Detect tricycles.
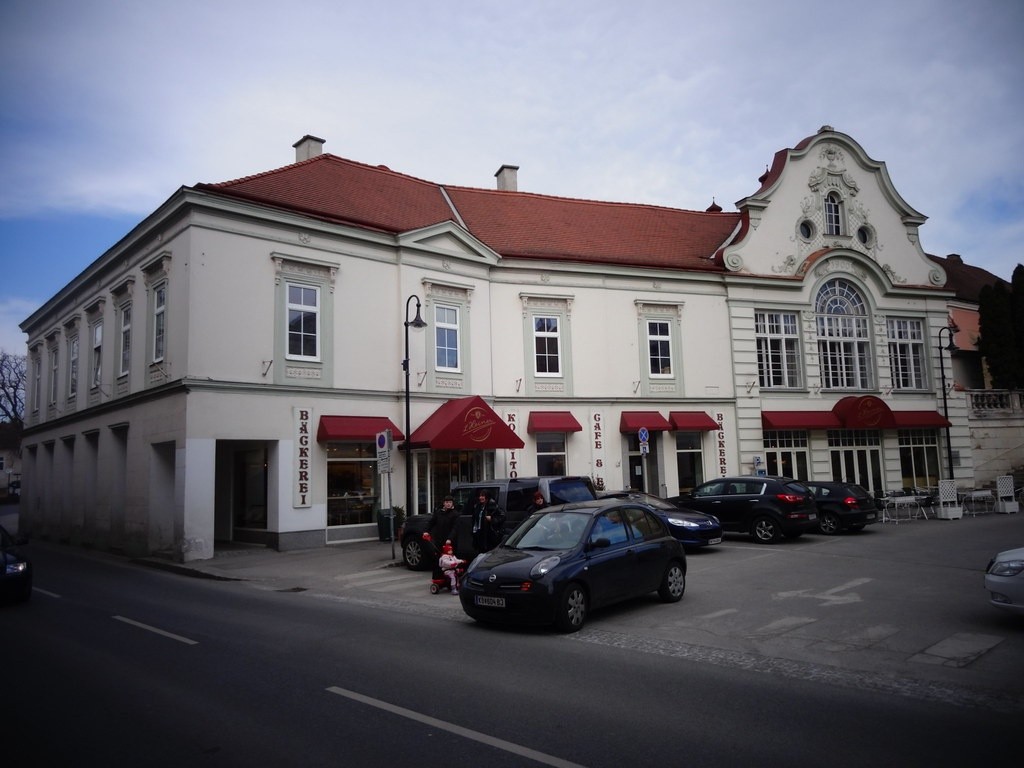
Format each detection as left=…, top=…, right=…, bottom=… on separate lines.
left=420, top=532, right=468, bottom=595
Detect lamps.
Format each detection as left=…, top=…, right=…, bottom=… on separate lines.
left=879, top=387, right=894, bottom=400
left=806, top=387, right=823, bottom=398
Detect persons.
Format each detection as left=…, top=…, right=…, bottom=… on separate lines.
left=528, top=492, right=552, bottom=520
left=429, top=488, right=505, bottom=595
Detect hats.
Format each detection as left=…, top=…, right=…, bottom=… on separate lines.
left=534, top=492, right=542, bottom=501
left=443, top=545, right=452, bottom=554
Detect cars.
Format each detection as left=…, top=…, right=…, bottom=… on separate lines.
left=803, top=480, right=879, bottom=537
left=456, top=497, right=687, bottom=635
left=982, top=547, right=1024, bottom=615
left=596, top=490, right=724, bottom=547
left=0, top=522, right=35, bottom=606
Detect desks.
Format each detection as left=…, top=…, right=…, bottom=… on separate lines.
left=869, top=486, right=997, bottom=525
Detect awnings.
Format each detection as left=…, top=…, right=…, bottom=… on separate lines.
left=761, top=395, right=952, bottom=430
left=317, top=415, right=405, bottom=442
left=526, top=411, right=583, bottom=433
left=398, top=395, right=525, bottom=451
left=619, top=411, right=720, bottom=433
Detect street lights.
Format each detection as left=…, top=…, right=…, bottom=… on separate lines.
left=938, top=325, right=960, bottom=479
left=400, top=292, right=430, bottom=518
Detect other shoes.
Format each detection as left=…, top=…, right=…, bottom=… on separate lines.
left=452, top=590, right=459, bottom=595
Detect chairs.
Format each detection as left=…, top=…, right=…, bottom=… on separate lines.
left=873, top=491, right=895, bottom=521
left=544, top=519, right=591, bottom=545
left=916, top=496, right=935, bottom=521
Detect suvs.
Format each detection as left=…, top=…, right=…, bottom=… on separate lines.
left=397, top=474, right=600, bottom=572
left=664, top=475, right=823, bottom=544
